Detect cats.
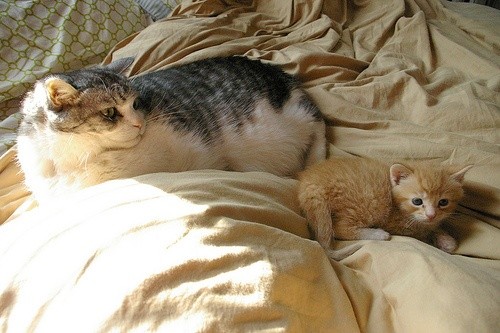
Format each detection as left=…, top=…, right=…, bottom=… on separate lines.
left=297, top=156, right=473, bottom=257
left=15, top=55, right=328, bottom=192
